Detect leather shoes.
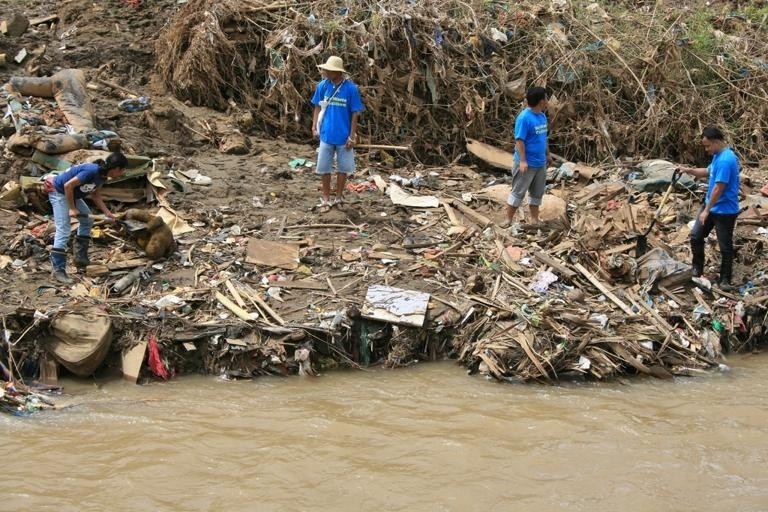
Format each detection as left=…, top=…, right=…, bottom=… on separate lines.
left=334, top=197, right=349, bottom=211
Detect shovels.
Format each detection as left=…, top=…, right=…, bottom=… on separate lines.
left=76, top=213, right=149, bottom=232
left=636, top=169, right=682, bottom=258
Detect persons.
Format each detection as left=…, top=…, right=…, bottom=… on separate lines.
left=47, top=153, right=129, bottom=285
left=677, top=125, right=741, bottom=285
left=310, top=54, right=365, bottom=208
left=501, top=87, right=550, bottom=229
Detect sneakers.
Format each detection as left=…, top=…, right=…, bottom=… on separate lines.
left=687, top=268, right=702, bottom=277
left=718, top=277, right=731, bottom=292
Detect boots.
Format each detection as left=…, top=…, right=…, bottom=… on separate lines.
left=49, top=249, right=75, bottom=284
left=72, top=235, right=103, bottom=266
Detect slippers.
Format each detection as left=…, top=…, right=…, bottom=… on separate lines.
left=320, top=200, right=332, bottom=213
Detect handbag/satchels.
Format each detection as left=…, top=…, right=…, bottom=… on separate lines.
left=315, top=100, right=328, bottom=136
left=42, top=177, right=55, bottom=192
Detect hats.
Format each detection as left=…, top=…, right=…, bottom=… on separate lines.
left=316, top=56, right=346, bottom=75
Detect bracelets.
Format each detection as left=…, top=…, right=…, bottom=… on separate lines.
left=348, top=135, right=355, bottom=143
